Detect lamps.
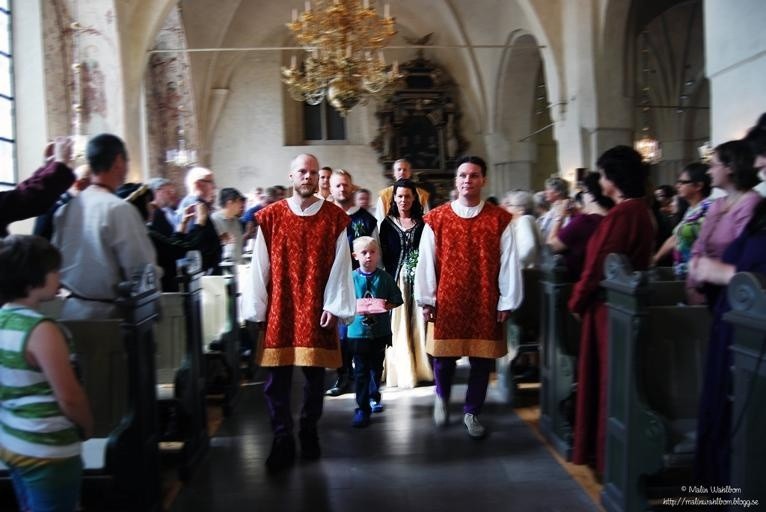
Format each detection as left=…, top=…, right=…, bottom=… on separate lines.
left=281, top=0, right=403, bottom=116
left=165, top=33, right=198, bottom=167
left=633, top=27, right=663, bottom=165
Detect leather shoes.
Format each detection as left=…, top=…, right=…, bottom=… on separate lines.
left=323, top=367, right=353, bottom=397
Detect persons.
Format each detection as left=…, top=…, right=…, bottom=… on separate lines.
left=0, top=109, right=765, bottom=512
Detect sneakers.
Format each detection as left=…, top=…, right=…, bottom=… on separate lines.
left=298, top=425, right=323, bottom=462
left=432, top=391, right=449, bottom=428
left=352, top=407, right=371, bottom=427
left=369, top=398, right=383, bottom=413
left=264, top=431, right=297, bottom=474
left=463, top=413, right=485, bottom=438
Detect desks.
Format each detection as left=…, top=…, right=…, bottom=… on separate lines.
left=724, top=272, right=766, bottom=512
left=538, top=245, right=582, bottom=461
left=601, top=252, right=707, bottom=511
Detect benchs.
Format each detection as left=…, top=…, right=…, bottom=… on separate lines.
left=0, top=268, right=160, bottom=511
left=155, top=249, right=211, bottom=481
left=201, top=261, right=242, bottom=415
